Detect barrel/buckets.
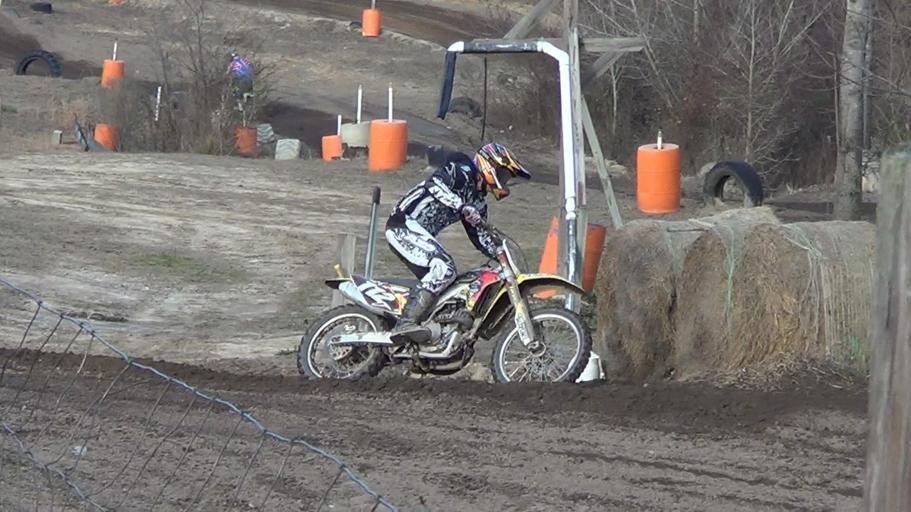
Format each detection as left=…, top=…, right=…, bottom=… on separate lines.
left=637, top=142, right=680, bottom=214
left=234, top=125, right=257, bottom=156
left=532, top=215, right=607, bottom=299
left=322, top=134, right=342, bottom=161
left=94, top=122, right=120, bottom=150
left=369, top=119, right=407, bottom=170
left=360, top=7, right=380, bottom=37
left=101, top=58, right=122, bottom=88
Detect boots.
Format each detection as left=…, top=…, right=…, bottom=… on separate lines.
left=390, top=289, right=438, bottom=343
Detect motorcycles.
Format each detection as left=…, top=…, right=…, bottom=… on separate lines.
left=296, top=185, right=596, bottom=387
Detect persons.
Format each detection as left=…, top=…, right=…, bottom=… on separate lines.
left=222, top=51, right=258, bottom=105
left=384, top=141, right=535, bottom=346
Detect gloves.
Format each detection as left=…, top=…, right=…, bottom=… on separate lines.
left=462, top=205, right=482, bottom=225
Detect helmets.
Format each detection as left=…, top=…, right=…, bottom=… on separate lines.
left=475, top=143, right=531, bottom=200
